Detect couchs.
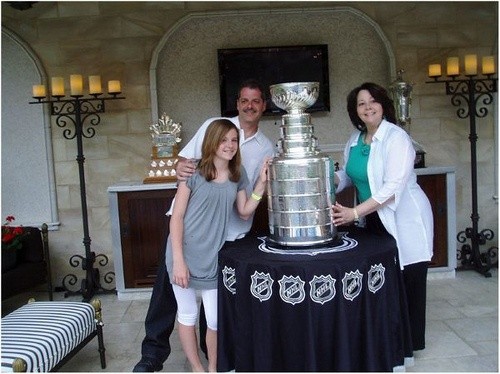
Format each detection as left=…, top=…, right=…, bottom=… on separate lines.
left=1, top=223, right=52, bottom=301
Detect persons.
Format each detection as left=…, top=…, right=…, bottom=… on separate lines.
left=132, top=83, right=279, bottom=373
left=328, top=82, right=434, bottom=350
left=165, top=119, right=272, bottom=373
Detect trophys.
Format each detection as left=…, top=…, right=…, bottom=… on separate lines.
left=267, top=81, right=337, bottom=247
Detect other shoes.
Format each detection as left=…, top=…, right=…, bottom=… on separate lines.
left=133, top=359, right=163, bottom=372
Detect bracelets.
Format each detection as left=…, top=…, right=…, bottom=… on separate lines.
left=251, top=192, right=262, bottom=201
left=353, top=208, right=359, bottom=221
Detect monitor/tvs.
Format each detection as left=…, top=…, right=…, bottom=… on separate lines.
left=218, top=44, right=330, bottom=119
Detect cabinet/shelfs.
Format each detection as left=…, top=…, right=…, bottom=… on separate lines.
left=107, top=165, right=456, bottom=300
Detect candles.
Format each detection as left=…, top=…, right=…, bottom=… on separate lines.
left=464, top=54, right=477, bottom=74
left=51, top=76, right=65, bottom=94
left=88, top=76, right=102, bottom=93
left=32, top=85, right=46, bottom=96
left=69, top=74, right=83, bottom=94
left=447, top=57, right=459, bottom=74
left=429, top=64, right=441, bottom=75
left=107, top=80, right=120, bottom=92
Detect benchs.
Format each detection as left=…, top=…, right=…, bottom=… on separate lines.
left=1, top=293, right=107, bottom=372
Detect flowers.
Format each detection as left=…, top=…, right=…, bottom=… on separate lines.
left=2, top=216, right=25, bottom=250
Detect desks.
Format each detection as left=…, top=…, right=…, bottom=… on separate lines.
left=217, top=227, right=415, bottom=373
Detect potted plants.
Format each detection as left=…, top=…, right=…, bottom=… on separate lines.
left=482, top=56, right=495, bottom=73
